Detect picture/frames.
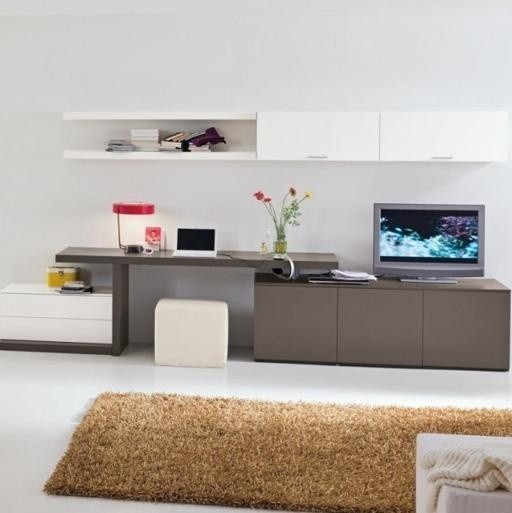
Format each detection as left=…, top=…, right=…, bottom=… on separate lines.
left=145, top=226, right=161, bottom=252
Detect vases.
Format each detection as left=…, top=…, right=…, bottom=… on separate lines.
left=273, top=233, right=288, bottom=260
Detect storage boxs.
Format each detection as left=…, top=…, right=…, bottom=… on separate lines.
left=47, top=266, right=80, bottom=289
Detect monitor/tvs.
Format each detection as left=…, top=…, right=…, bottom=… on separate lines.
left=372, top=201, right=486, bottom=284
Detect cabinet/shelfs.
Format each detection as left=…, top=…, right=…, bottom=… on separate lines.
left=380, top=109, right=511, bottom=167
left=1, top=283, right=112, bottom=344
left=250, top=270, right=511, bottom=375
left=255, top=109, right=380, bottom=166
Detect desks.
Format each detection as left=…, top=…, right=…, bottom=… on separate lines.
left=54, top=247, right=340, bottom=356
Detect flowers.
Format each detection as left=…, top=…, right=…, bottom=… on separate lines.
left=253, top=188, right=313, bottom=253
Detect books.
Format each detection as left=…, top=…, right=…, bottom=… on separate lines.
left=130, top=129, right=158, bottom=152
left=107, top=138, right=134, bottom=153
left=56, top=279, right=97, bottom=297
left=329, top=269, right=379, bottom=282
left=159, top=131, right=210, bottom=151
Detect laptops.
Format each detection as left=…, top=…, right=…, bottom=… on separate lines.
left=174, top=227, right=218, bottom=257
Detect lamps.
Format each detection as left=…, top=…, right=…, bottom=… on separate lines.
left=112, top=201, right=156, bottom=254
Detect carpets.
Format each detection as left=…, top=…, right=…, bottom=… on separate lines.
left=43, top=392, right=512, bottom=513
